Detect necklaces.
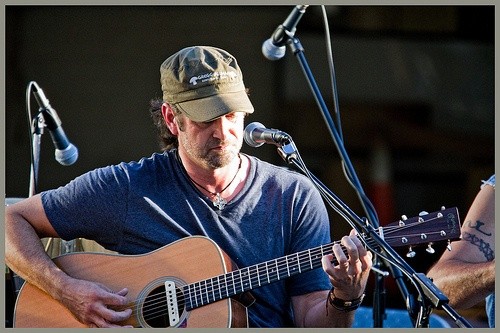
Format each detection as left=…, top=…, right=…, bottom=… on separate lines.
left=177, top=152, right=241, bottom=209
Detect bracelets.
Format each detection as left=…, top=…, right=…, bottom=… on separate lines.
left=326, top=287, right=365, bottom=317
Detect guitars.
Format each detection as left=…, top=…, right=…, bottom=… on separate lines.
left=12, top=205, right=463, bottom=328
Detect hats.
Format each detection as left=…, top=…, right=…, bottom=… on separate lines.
left=161, top=46, right=255, bottom=123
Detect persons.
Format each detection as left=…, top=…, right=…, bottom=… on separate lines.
left=5, top=46, right=372, bottom=329
left=426, top=175, right=495, bottom=328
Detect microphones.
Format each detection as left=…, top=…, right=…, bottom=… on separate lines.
left=261, top=5, right=309, bottom=61
left=31, top=82, right=80, bottom=167
left=243, top=122, right=290, bottom=148
